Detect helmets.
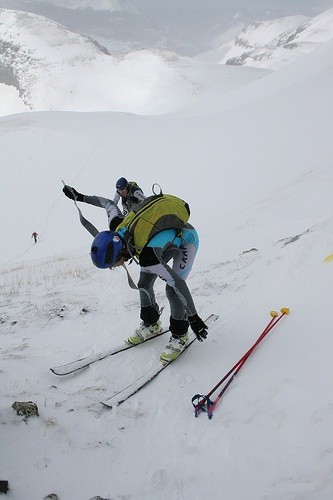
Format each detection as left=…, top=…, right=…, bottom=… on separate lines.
left=91, top=231, right=124, bottom=269
left=116, top=177, right=131, bottom=188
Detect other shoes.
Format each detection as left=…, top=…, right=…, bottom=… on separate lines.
left=128, top=320, right=162, bottom=345
left=159, top=335, right=190, bottom=364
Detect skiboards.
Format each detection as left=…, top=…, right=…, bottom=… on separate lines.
left=50, top=314, right=218, bottom=408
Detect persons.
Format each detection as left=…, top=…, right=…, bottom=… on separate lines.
left=113, top=177, right=145, bottom=216
left=32, top=232, right=38, bottom=243
left=63, top=185, right=209, bottom=361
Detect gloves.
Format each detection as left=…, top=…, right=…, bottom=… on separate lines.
left=188, top=314, right=209, bottom=342
left=62, top=186, right=85, bottom=202
